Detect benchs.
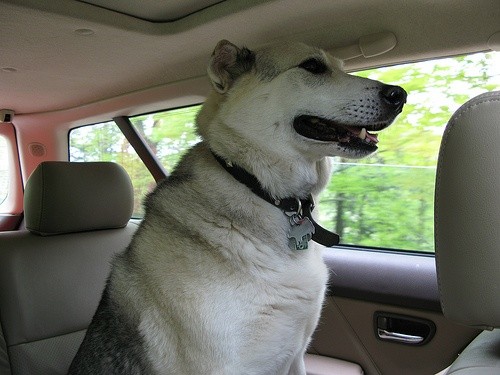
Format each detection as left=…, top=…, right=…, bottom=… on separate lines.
left=0, top=161, right=365, bottom=375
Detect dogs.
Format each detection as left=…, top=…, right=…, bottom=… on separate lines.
left=65, top=38, right=407, bottom=375
